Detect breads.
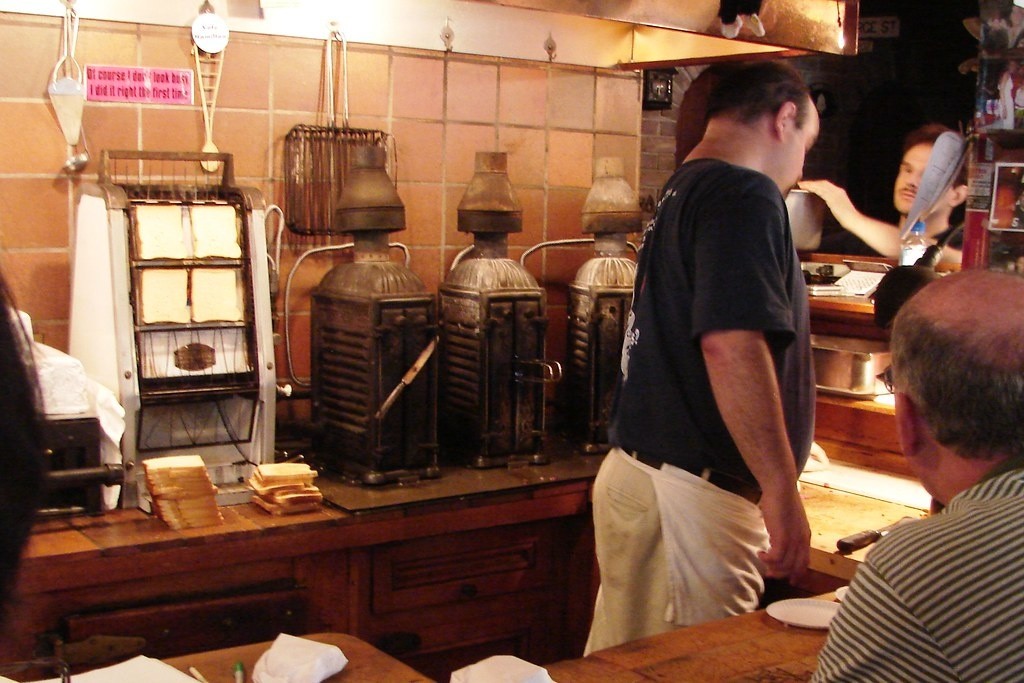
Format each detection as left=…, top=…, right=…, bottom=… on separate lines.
left=246, top=463, right=324, bottom=515
left=142, top=454, right=225, bottom=530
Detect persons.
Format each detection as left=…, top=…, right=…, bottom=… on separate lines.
left=809, top=271, right=1024, bottom=683
left=1, top=277, right=44, bottom=683
left=582, top=62, right=971, bottom=657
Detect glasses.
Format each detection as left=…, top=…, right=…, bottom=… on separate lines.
left=875, top=364, right=911, bottom=394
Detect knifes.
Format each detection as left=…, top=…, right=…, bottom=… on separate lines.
left=837, top=516, right=920, bottom=555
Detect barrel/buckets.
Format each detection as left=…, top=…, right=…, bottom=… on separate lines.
left=784, top=189, right=826, bottom=251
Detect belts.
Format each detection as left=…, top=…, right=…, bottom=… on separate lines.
left=617, top=446, right=765, bottom=504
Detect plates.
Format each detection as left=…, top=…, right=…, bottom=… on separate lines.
left=766, top=599, right=840, bottom=629
left=835, top=586, right=850, bottom=601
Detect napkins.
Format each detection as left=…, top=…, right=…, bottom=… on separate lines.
left=450, top=655, right=558, bottom=683
left=251, top=633, right=349, bottom=683
